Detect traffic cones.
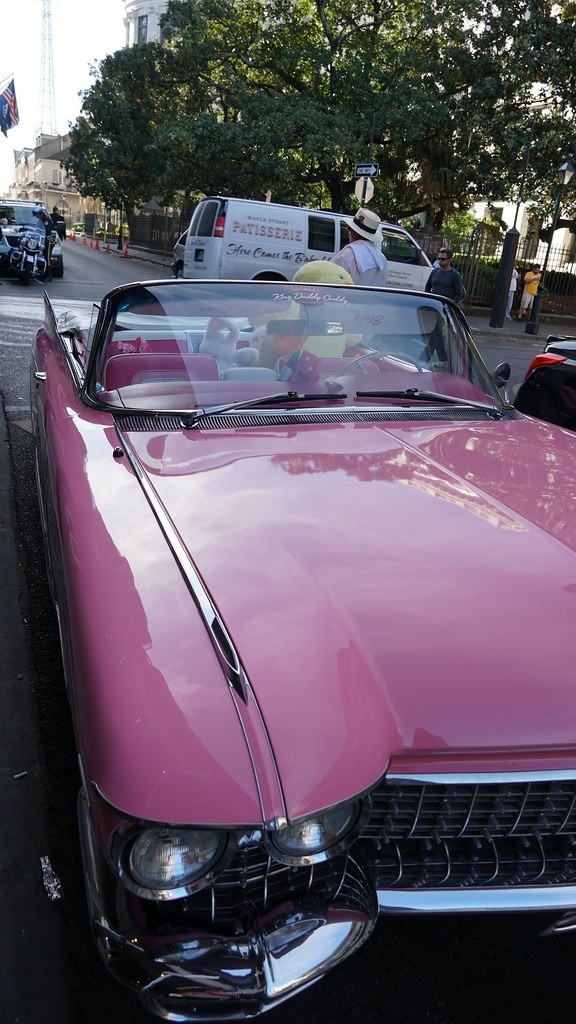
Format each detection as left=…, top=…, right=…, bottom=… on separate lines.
left=106, top=243, right=110, bottom=253
left=89, top=239, right=94, bottom=249
left=72, top=232, right=76, bottom=241
left=83, top=237, right=88, bottom=245
left=122, top=245, right=129, bottom=258
left=94, top=237, right=100, bottom=250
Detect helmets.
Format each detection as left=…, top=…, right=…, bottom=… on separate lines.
left=32, top=207, right=48, bottom=221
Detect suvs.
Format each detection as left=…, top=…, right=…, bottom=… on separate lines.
left=0, top=198, right=64, bottom=279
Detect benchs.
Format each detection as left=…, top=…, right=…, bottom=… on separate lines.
left=106, top=331, right=260, bottom=365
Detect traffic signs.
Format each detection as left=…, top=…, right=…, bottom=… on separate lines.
left=355, top=164, right=378, bottom=178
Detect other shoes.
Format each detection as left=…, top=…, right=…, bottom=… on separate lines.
left=40, top=276, right=53, bottom=282
left=417, top=361, right=428, bottom=369
left=505, top=316, right=513, bottom=321
left=434, top=361, right=449, bottom=367
left=517, top=317, right=522, bottom=323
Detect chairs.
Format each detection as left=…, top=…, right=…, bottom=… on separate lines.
left=275, top=356, right=380, bottom=384
left=104, top=353, right=221, bottom=392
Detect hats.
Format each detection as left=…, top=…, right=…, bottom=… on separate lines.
left=344, top=207, right=382, bottom=241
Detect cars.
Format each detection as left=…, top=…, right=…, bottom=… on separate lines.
left=170, top=228, right=190, bottom=278
left=32, top=279, right=576, bottom=1024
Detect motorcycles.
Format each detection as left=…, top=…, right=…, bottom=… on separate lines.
left=11, top=228, right=56, bottom=287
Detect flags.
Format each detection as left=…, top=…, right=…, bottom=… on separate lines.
left=0, top=80, right=20, bottom=137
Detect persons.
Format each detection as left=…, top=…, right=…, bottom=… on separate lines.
left=50, top=207, right=66, bottom=241
left=33, top=207, right=57, bottom=282
left=333, top=208, right=388, bottom=287
left=505, top=263, right=541, bottom=322
left=418, top=247, right=463, bottom=368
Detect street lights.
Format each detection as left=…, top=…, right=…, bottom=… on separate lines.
left=525, top=153, right=575, bottom=335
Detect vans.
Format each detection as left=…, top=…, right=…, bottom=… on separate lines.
left=184, top=197, right=434, bottom=293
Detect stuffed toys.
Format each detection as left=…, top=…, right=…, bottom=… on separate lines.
left=247, top=260, right=363, bottom=369
left=201, top=317, right=259, bottom=375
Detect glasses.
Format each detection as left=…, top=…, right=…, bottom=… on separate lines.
left=346, top=226, right=358, bottom=236
left=438, top=257, right=449, bottom=261
